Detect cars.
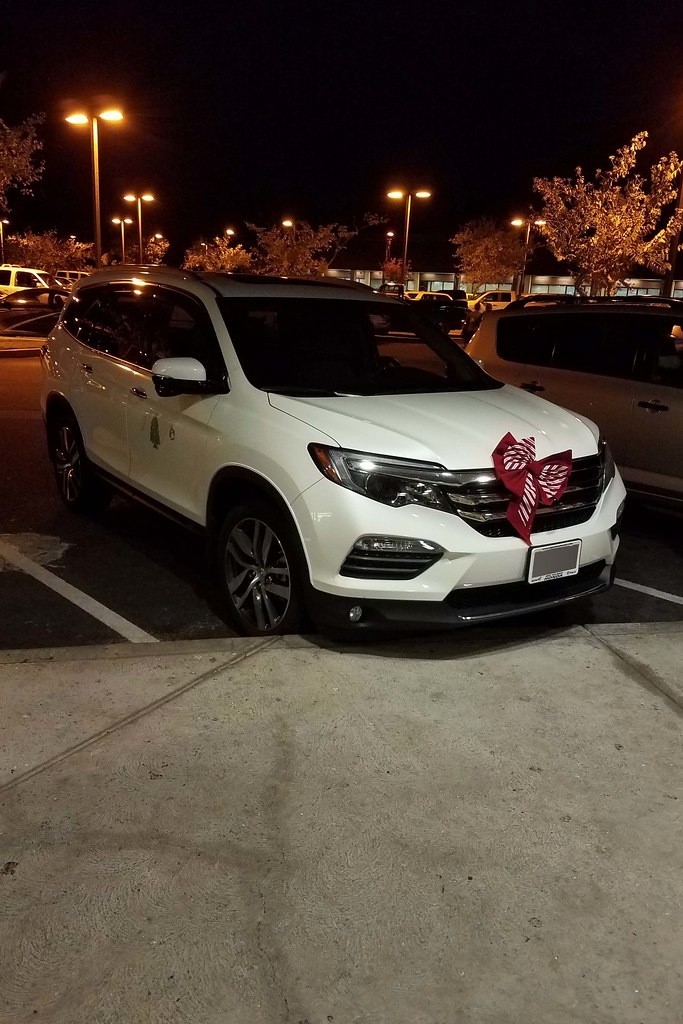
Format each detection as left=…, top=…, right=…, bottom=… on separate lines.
left=57, top=276, right=77, bottom=288
left=0, top=288, right=70, bottom=352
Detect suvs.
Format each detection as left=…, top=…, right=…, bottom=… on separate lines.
left=0, top=264, right=78, bottom=306
left=37, top=261, right=630, bottom=635
left=462, top=294, right=683, bottom=519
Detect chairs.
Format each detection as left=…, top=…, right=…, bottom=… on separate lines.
left=312, top=314, right=378, bottom=395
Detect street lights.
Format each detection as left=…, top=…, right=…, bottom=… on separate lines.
left=0, top=220, right=10, bottom=265
left=124, top=193, right=156, bottom=264
left=282, top=219, right=296, bottom=238
left=64, top=110, right=124, bottom=269
left=387, top=191, right=431, bottom=284
left=509, top=219, right=546, bottom=281
left=111, top=218, right=134, bottom=264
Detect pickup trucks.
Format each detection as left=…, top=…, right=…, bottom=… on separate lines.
left=466, top=290, right=558, bottom=316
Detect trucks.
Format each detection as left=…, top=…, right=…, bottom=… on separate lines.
left=377, top=284, right=468, bottom=316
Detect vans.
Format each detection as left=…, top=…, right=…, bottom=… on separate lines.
left=405, top=286, right=466, bottom=302
left=55, top=270, right=91, bottom=279
